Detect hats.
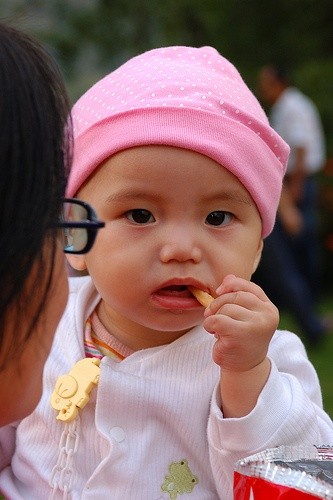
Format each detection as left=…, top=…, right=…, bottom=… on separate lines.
left=61, top=45, right=292, bottom=241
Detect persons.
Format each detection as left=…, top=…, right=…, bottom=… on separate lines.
left=0, top=44, right=333, bottom=500
left=251, top=54, right=333, bottom=352
left=0, top=21, right=107, bottom=428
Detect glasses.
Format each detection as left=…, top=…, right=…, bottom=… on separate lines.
left=55, top=196, right=107, bottom=255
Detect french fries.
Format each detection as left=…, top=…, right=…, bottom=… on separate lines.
left=186, top=285, right=215, bottom=309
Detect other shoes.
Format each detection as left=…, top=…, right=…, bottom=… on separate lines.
left=306, top=319, right=332, bottom=350
